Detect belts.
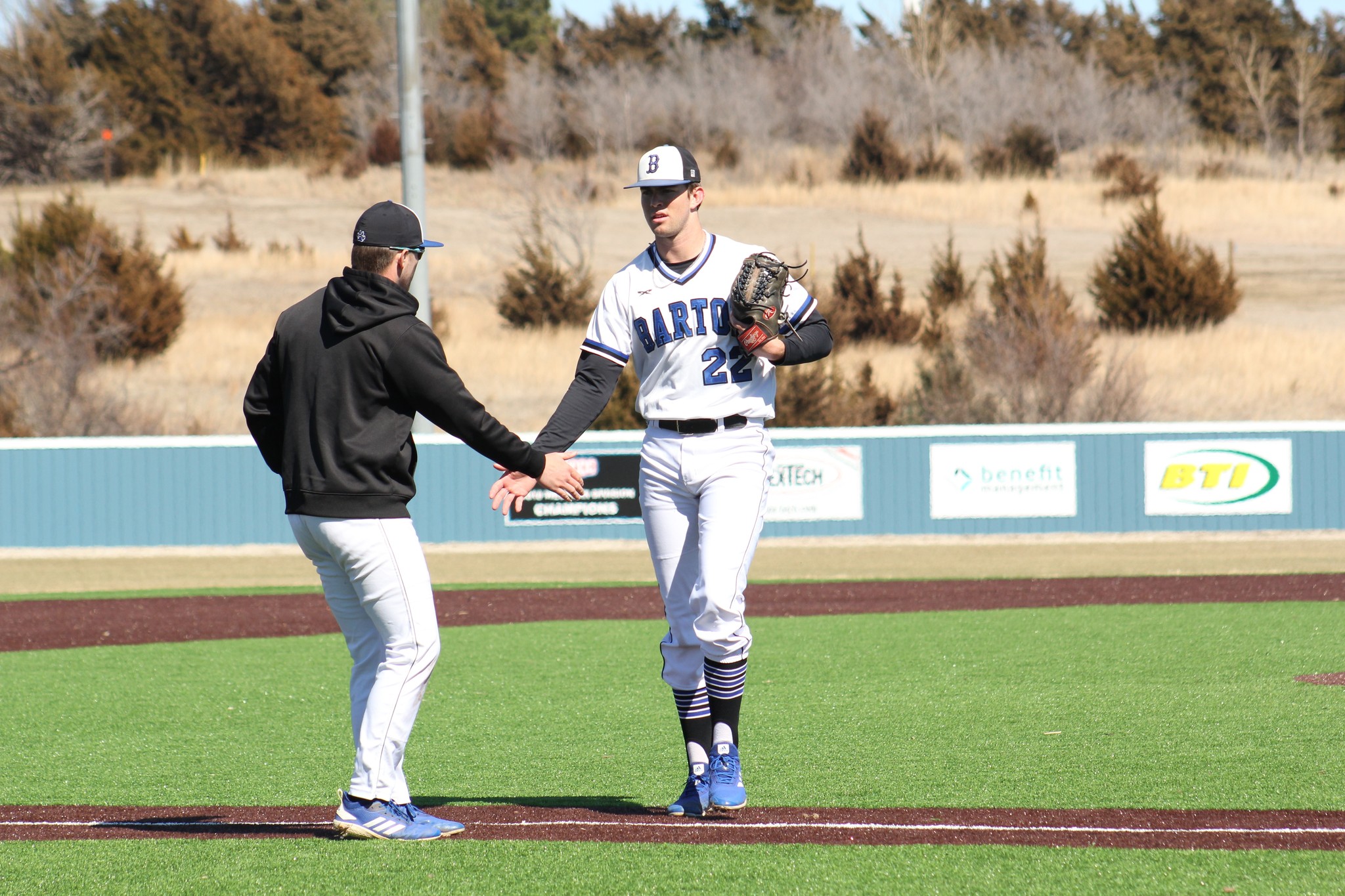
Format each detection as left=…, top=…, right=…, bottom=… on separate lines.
left=646, top=413, right=747, bottom=436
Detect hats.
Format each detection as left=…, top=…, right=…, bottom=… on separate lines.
left=353, top=200, right=444, bottom=247
left=623, top=144, right=701, bottom=189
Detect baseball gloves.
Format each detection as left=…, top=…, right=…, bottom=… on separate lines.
left=728, top=250, right=809, bottom=357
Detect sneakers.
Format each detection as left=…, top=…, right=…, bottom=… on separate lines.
left=667, top=762, right=713, bottom=817
left=333, top=788, right=441, bottom=841
left=708, top=742, right=747, bottom=810
left=392, top=803, right=465, bottom=837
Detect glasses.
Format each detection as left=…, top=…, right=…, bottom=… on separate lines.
left=390, top=246, right=425, bottom=260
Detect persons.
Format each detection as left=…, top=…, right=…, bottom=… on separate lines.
left=488, top=144, right=833, bottom=816
left=243, top=200, right=584, bottom=841
